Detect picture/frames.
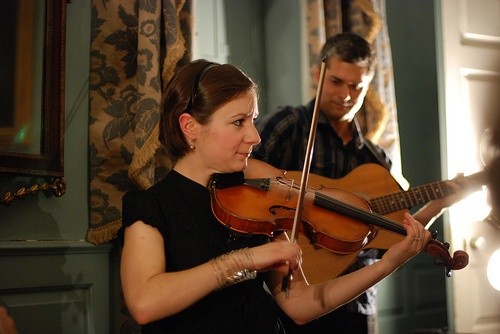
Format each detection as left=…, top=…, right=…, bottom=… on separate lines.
left=0, top=0, right=68, bottom=178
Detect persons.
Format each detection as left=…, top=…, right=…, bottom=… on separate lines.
left=249, top=31, right=484, bottom=334
left=119, top=59, right=431, bottom=334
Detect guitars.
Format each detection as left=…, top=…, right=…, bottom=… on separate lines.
left=274, top=163, right=500, bottom=288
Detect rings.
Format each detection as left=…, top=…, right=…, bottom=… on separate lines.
left=414, top=238, right=421, bottom=240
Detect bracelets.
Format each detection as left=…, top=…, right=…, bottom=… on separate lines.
left=211, top=247, right=257, bottom=290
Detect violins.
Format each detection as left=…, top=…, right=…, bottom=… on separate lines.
left=210, top=158, right=469, bottom=278
